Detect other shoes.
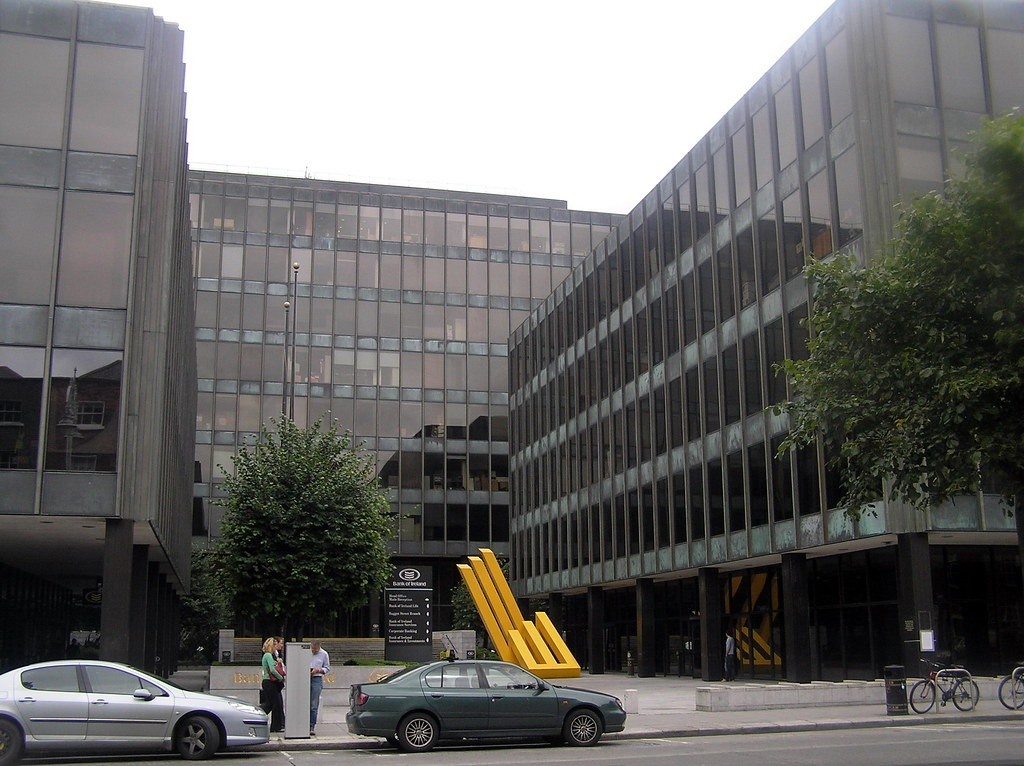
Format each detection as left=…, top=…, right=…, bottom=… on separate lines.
left=270, top=729, right=286, bottom=732
left=721, top=678, right=726, bottom=682
left=310, top=727, right=315, bottom=734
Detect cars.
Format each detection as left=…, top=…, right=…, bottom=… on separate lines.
left=0, top=660, right=270, bottom=765
left=347, top=650, right=628, bottom=754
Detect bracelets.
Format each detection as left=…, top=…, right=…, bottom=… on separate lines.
left=319, top=669, right=320, bottom=672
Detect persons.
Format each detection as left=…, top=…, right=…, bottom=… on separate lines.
left=309, top=639, right=330, bottom=735
left=66, top=638, right=96, bottom=660
left=720, top=631, right=735, bottom=683
left=255, top=636, right=286, bottom=732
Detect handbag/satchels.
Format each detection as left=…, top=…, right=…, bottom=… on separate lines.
left=266, top=653, right=287, bottom=681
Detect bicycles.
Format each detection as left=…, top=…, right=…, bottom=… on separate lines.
left=909, top=657, right=980, bottom=715
left=997, top=663, right=1024, bottom=710
